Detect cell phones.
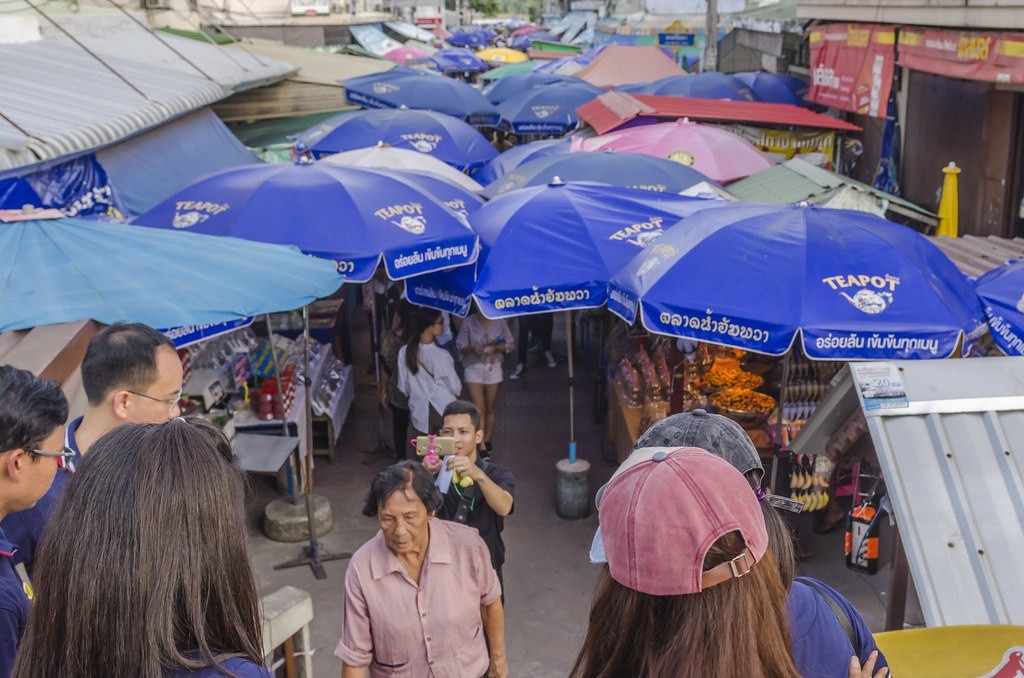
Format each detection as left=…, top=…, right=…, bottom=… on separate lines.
left=416, top=436, right=455, bottom=456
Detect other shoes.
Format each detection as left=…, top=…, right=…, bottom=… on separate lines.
left=479, top=451, right=491, bottom=461
left=484, top=441, right=493, bottom=453
left=528, top=336, right=538, bottom=351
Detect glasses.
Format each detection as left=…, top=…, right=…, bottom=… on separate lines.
left=434, top=317, right=445, bottom=326
left=0, top=436, right=77, bottom=471
left=126, top=387, right=182, bottom=412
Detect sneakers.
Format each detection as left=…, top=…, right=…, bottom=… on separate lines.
left=509, top=362, right=525, bottom=380
left=543, top=350, right=557, bottom=368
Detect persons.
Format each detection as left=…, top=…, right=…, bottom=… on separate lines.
left=0, top=320, right=183, bottom=578
left=509, top=315, right=557, bottom=380
left=10, top=416, right=269, bottom=678
left=456, top=313, right=516, bottom=460
left=434, top=312, right=461, bottom=375
left=565, top=446, right=889, bottom=678
left=0, top=362, right=77, bottom=678
left=595, top=409, right=889, bottom=678
left=380, top=301, right=419, bottom=459
left=362, top=275, right=400, bottom=374
left=421, top=400, right=515, bottom=656
left=396, top=307, right=463, bottom=463
left=333, top=460, right=510, bottom=678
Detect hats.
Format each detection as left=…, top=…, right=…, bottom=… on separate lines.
left=632, top=408, right=764, bottom=490
left=589, top=447, right=767, bottom=597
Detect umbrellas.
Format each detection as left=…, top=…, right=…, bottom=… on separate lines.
left=132, top=24, right=1024, bottom=556
left=1, top=204, right=347, bottom=333
